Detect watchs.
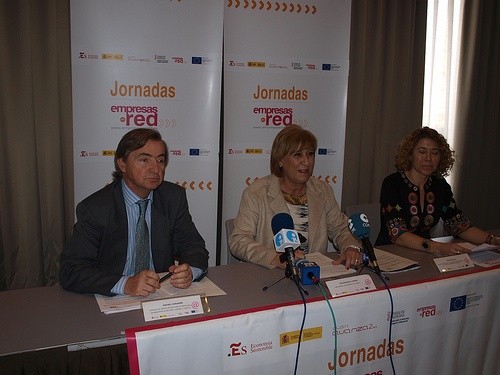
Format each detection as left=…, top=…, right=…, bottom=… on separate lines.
left=421, top=239, right=431, bottom=253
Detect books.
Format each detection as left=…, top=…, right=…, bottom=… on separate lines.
left=338, top=247, right=420, bottom=275
left=94, top=272, right=227, bottom=316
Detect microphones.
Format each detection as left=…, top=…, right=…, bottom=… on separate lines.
left=348, top=212, right=381, bottom=276
left=271, top=213, right=301, bottom=285
left=295, top=259, right=320, bottom=285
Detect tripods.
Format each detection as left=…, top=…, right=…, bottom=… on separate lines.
left=263, top=263, right=309, bottom=296
left=358, top=252, right=390, bottom=280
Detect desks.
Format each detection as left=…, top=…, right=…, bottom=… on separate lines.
left=0, top=228, right=500, bottom=375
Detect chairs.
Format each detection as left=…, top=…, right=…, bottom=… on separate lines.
left=225, top=218, right=240, bottom=264
left=344, top=203, right=380, bottom=247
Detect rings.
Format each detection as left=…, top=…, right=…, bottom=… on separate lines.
left=356, top=260, right=359, bottom=263
left=458, top=251, right=459, bottom=254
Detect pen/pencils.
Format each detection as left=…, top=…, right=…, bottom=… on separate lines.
left=380, top=273, right=389, bottom=280
left=158, top=270, right=174, bottom=283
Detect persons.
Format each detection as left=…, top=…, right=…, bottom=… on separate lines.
left=373, top=127, right=500, bottom=256
left=227, top=125, right=365, bottom=271
left=58, top=128, right=210, bottom=298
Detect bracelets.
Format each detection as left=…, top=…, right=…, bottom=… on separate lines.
left=484, top=232, right=496, bottom=245
left=349, top=245, right=360, bottom=252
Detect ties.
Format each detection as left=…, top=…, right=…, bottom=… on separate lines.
left=134, top=199, right=150, bottom=276
